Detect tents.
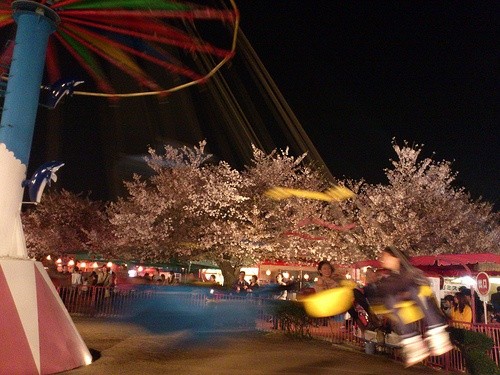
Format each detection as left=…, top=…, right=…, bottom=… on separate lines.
left=353, top=253, right=499, bottom=324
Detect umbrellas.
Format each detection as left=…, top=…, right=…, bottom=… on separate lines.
left=261, top=257, right=351, bottom=290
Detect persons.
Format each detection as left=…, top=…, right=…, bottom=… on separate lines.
left=365, top=239, right=455, bottom=363
left=315, top=262, right=383, bottom=334
left=60, top=262, right=317, bottom=335
left=434, top=286, right=499, bottom=326
